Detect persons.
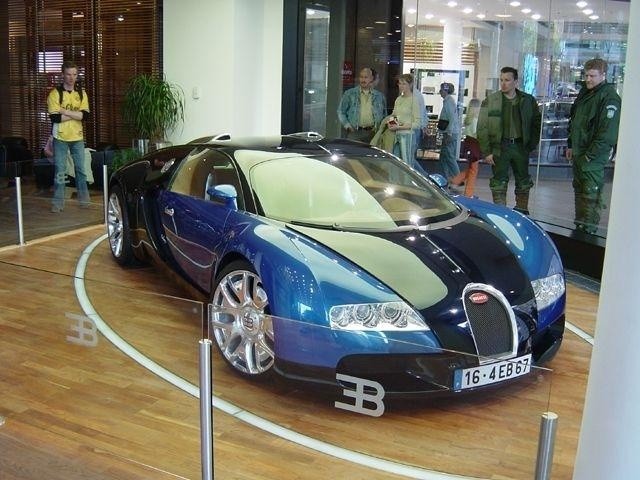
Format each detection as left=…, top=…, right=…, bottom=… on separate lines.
left=438, top=83, right=465, bottom=185
left=565, top=65, right=585, bottom=98
left=450, top=98, right=482, bottom=198
left=33, top=137, right=54, bottom=196
left=370, top=67, right=428, bottom=177
left=566, top=58, right=621, bottom=236
left=337, top=68, right=385, bottom=142
left=47, top=62, right=97, bottom=213
left=476, top=67, right=541, bottom=212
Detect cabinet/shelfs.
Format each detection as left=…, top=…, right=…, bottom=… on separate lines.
left=416, top=68, right=468, bottom=150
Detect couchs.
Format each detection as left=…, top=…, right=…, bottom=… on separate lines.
left=35, top=146, right=122, bottom=187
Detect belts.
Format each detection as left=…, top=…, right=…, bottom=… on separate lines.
left=359, top=125, right=374, bottom=131
left=502, top=138, right=521, bottom=145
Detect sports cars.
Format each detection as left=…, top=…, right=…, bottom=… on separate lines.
left=106, top=132, right=568, bottom=397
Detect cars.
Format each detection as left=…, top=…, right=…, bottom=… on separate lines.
left=533, top=101, right=571, bottom=139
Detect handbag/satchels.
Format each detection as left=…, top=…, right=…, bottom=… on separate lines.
left=437, top=119, right=449, bottom=130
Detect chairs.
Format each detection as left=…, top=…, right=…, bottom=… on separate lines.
left=0, top=134, right=31, bottom=177
left=417, top=127, right=436, bottom=156
left=547, top=126, right=569, bottom=160
